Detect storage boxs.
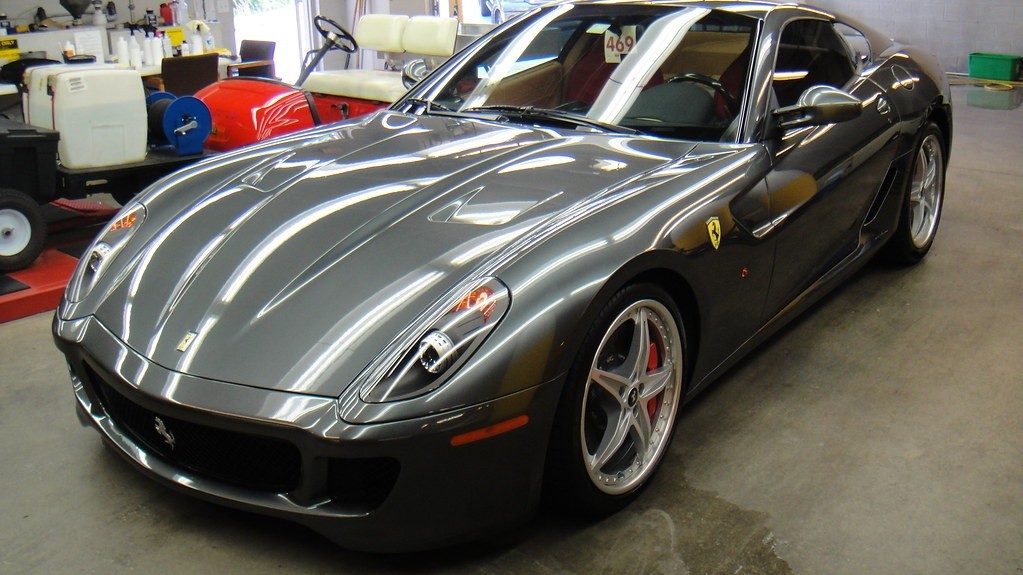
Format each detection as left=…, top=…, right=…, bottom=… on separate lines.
left=968, top=52, right=1023, bottom=87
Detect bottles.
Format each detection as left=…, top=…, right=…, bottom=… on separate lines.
left=112, top=23, right=214, bottom=69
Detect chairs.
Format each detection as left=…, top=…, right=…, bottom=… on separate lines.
left=566, top=31, right=664, bottom=108
left=222, top=39, right=276, bottom=80
left=712, top=24, right=829, bottom=134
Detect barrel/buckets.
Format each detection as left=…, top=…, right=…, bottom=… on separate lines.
left=18, top=61, right=149, bottom=168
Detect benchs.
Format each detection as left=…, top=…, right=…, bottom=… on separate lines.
left=300, top=13, right=460, bottom=105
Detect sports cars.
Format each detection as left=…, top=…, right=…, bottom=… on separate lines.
left=51, top=0, right=955, bottom=555
